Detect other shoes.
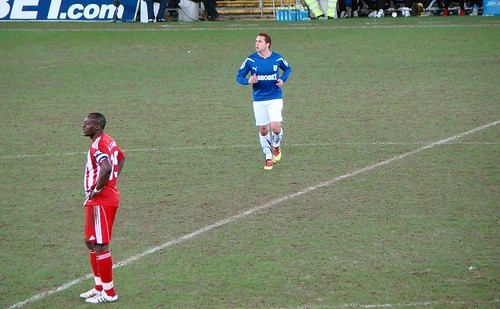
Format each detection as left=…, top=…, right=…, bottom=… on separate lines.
left=203, top=11, right=209, bottom=20
left=460, top=9, right=465, bottom=16
left=444, top=9, right=449, bottom=16
left=215, top=16, right=225, bottom=20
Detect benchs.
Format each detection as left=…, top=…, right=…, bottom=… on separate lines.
left=213, top=1, right=294, bottom=17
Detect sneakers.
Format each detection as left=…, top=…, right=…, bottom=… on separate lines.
left=264, top=160, right=276, bottom=170
left=148, top=19, right=153, bottom=23
left=340, top=11, right=348, bottom=18
left=274, top=147, right=281, bottom=160
left=80, top=288, right=119, bottom=303
left=159, top=18, right=166, bottom=22
left=353, top=11, right=358, bottom=17
left=367, top=9, right=385, bottom=18
left=399, top=7, right=412, bottom=17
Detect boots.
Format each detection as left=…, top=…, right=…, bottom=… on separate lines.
left=470, top=4, right=478, bottom=16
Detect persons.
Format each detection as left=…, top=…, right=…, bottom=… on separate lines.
left=416, top=0, right=437, bottom=15
left=143, top=0, right=169, bottom=23
left=466, top=0, right=484, bottom=16
left=338, top=0, right=359, bottom=18
left=236, top=33, right=292, bottom=171
left=190, top=0, right=227, bottom=21
left=79, top=112, right=126, bottom=304
left=392, top=0, right=415, bottom=18
left=443, top=0, right=465, bottom=16
left=362, top=0, right=385, bottom=18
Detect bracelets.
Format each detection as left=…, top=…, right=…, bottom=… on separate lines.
left=94, top=187, right=100, bottom=193
